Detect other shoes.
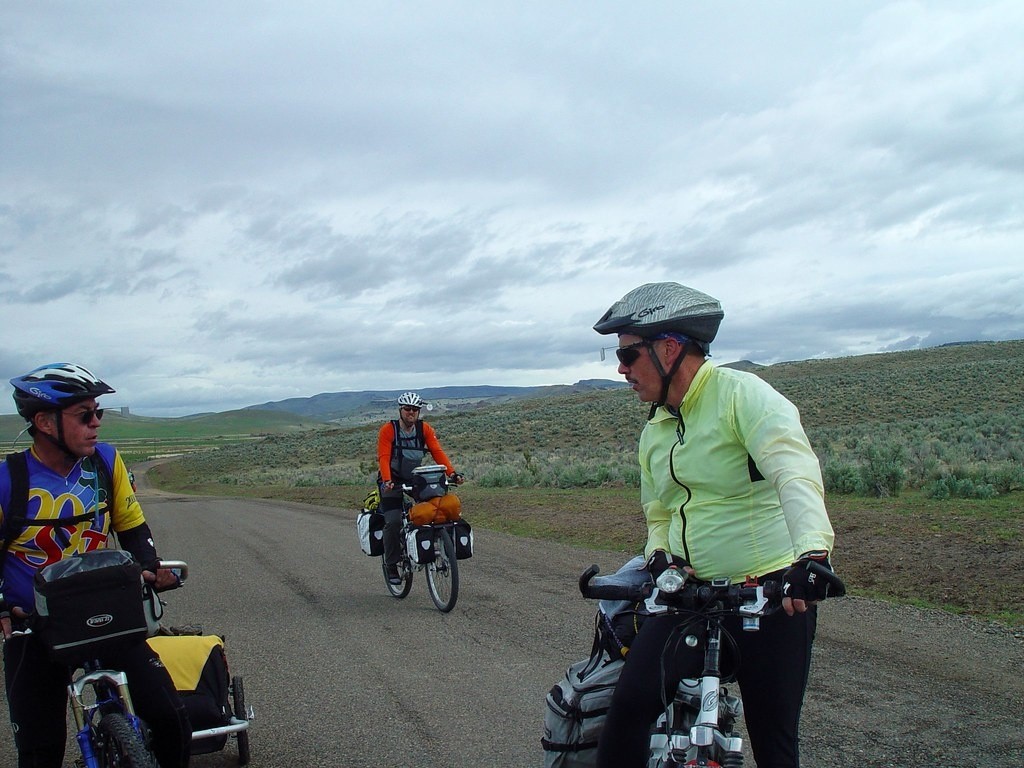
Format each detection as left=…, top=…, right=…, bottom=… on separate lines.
left=384, top=567, right=401, bottom=585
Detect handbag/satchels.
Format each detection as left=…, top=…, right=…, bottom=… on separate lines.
left=144, top=634, right=230, bottom=755
left=404, top=523, right=435, bottom=564
left=364, top=488, right=383, bottom=513
left=447, top=519, right=474, bottom=558
left=540, top=653, right=625, bottom=768
left=357, top=513, right=386, bottom=556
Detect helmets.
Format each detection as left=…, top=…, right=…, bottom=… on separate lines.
left=596, top=282, right=726, bottom=344
left=396, top=392, right=425, bottom=408
left=9, top=362, right=116, bottom=416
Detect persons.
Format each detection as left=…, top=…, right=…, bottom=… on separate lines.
left=595, top=281, right=836, bottom=768
left=127, top=468, right=134, bottom=485
left=376, top=392, right=465, bottom=585
left=0, top=363, right=180, bottom=768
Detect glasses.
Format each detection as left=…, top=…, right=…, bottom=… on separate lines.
left=616, top=337, right=660, bottom=366
left=400, top=404, right=420, bottom=411
left=61, top=409, right=103, bottom=423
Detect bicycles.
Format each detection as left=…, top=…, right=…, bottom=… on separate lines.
left=578, top=561, right=848, bottom=768
left=381, top=464, right=462, bottom=613
left=0, top=559, right=255, bottom=768
